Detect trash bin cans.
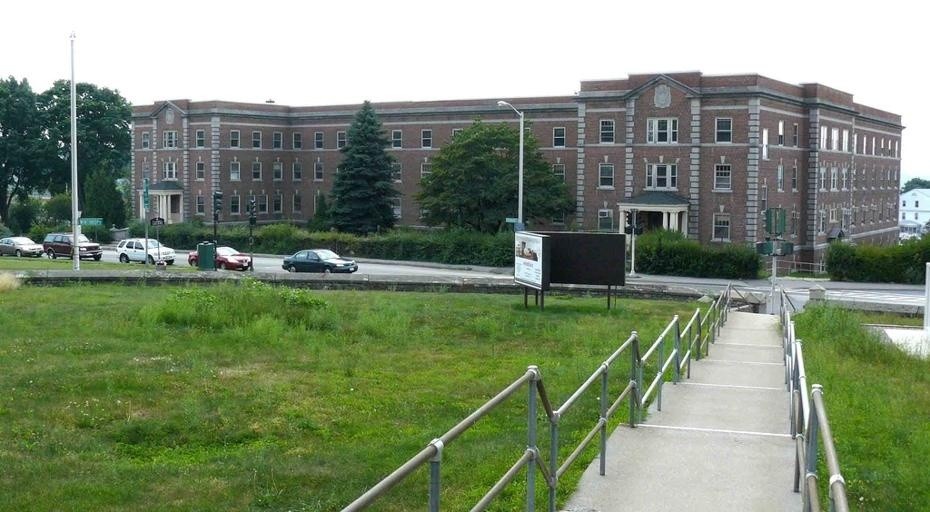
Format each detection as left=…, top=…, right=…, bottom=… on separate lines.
left=196, top=242, right=216, bottom=271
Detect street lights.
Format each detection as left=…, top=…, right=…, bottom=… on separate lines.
left=496, top=100, right=525, bottom=231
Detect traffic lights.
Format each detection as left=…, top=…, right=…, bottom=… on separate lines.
left=625, top=211, right=632, bottom=225
left=251, top=200, right=257, bottom=220
left=635, top=211, right=644, bottom=225
left=214, top=190, right=223, bottom=215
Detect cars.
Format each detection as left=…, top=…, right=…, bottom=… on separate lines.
left=188, top=246, right=251, bottom=270
left=116, top=237, right=176, bottom=265
left=281, top=248, right=358, bottom=274
left=42, top=232, right=102, bottom=262
left=0, top=236, right=44, bottom=258
